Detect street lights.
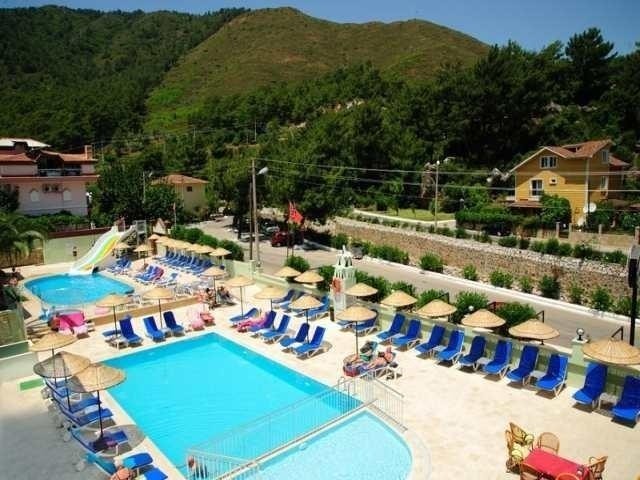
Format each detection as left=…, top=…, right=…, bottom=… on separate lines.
left=434, top=160, right=440, bottom=233
left=250, top=166, right=268, bottom=259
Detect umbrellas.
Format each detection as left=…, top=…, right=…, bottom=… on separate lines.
left=510, top=309, right=558, bottom=345
left=288, top=293, right=325, bottom=344
left=292, top=269, right=325, bottom=296
left=66, top=363, right=126, bottom=436
left=114, top=233, right=232, bottom=304
left=252, top=284, right=287, bottom=329
left=581, top=337, right=640, bottom=395
left=34, top=351, right=90, bottom=410
left=29, top=332, right=79, bottom=387
left=225, top=275, right=255, bottom=319
left=275, top=266, right=301, bottom=278
left=338, top=282, right=507, bottom=359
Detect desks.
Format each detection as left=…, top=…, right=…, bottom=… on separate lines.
left=432, top=344, right=447, bottom=360
left=475, top=356, right=491, bottom=372
left=526, top=369, right=546, bottom=387
left=390, top=332, right=405, bottom=345
left=598, top=392, right=619, bottom=412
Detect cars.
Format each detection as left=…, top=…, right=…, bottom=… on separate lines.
left=260, top=219, right=304, bottom=246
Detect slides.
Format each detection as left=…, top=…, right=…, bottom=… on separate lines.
left=69, top=224, right=137, bottom=275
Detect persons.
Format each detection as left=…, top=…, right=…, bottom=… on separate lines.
left=348, top=344, right=393, bottom=370
left=109, top=456, right=135, bottom=480
left=8, top=276, right=18, bottom=287
left=49, top=311, right=60, bottom=332
left=187, top=456, right=206, bottom=478
left=73, top=246, right=79, bottom=260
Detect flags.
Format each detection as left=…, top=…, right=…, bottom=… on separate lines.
left=289, top=202, right=302, bottom=224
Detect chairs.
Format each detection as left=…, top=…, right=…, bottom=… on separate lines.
left=336, top=320, right=355, bottom=329
left=377, top=313, right=406, bottom=343
left=281, top=322, right=311, bottom=351
left=295, top=325, right=327, bottom=361
left=343, top=342, right=378, bottom=376
left=163, top=311, right=185, bottom=337
left=415, top=325, right=447, bottom=359
left=354, top=307, right=380, bottom=337
left=120, top=318, right=141, bottom=346
left=41, top=374, right=169, bottom=480
left=261, top=313, right=292, bottom=344
left=534, top=353, right=569, bottom=399
left=505, top=421, right=608, bottom=480
left=230, top=307, right=259, bottom=327
left=272, top=289, right=330, bottom=320
left=248, top=310, right=277, bottom=336
left=610, top=375, right=640, bottom=425
left=571, top=360, right=610, bottom=411
left=143, top=316, right=163, bottom=340
left=508, top=344, right=541, bottom=386
left=358, top=350, right=396, bottom=381
left=438, top=329, right=468, bottom=365
left=482, top=339, right=514, bottom=380
left=393, top=317, right=423, bottom=350
left=109, top=254, right=225, bottom=299
left=459, top=335, right=486, bottom=372
